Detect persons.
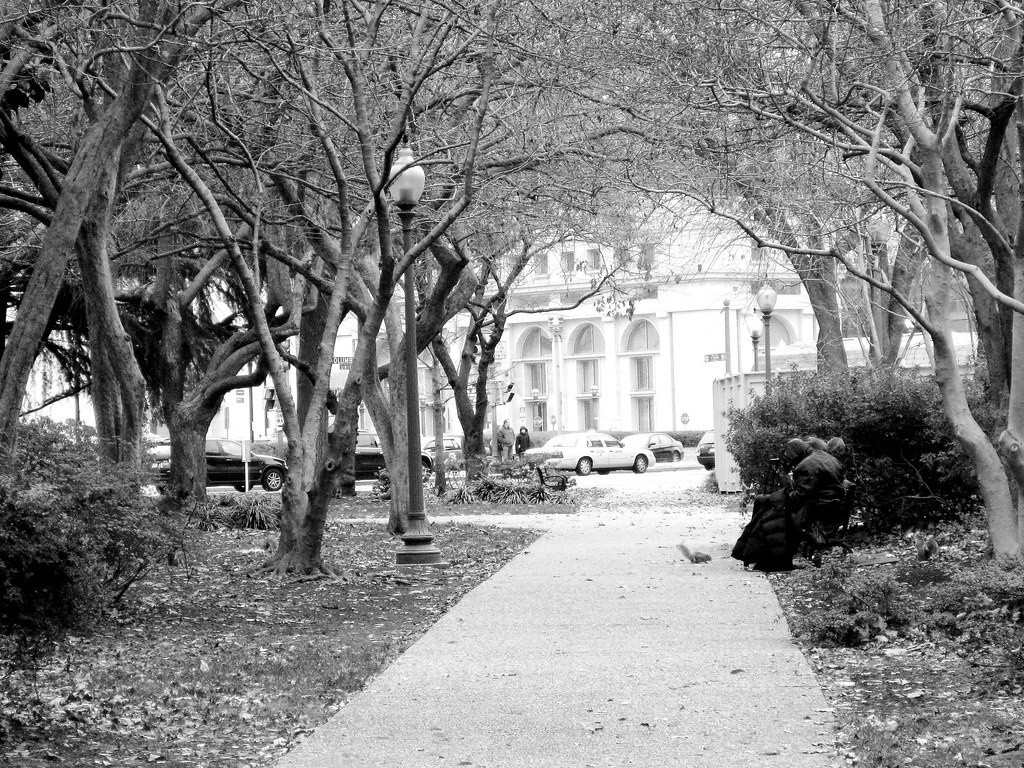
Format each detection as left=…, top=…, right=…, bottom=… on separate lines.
left=499, top=419, right=515, bottom=462
left=749, top=433, right=856, bottom=547
left=516, top=426, right=531, bottom=461
left=495, top=425, right=504, bottom=462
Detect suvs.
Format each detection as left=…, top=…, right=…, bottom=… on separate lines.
left=355, top=429, right=434, bottom=484
left=148, top=436, right=289, bottom=496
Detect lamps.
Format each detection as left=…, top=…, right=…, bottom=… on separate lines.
left=590, top=384, right=599, bottom=402
left=531, top=388, right=540, bottom=405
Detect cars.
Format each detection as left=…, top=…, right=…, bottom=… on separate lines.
left=421, top=434, right=491, bottom=464
left=620, top=433, right=684, bottom=463
left=696, top=430, right=715, bottom=470
left=523, top=430, right=657, bottom=476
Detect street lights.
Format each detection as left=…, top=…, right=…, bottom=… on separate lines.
left=745, top=307, right=765, bottom=372
left=388, top=132, right=444, bottom=565
left=757, top=274, right=777, bottom=396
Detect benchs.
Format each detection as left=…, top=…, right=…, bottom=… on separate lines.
left=801, top=480, right=857, bottom=565
left=536, top=465, right=569, bottom=491
